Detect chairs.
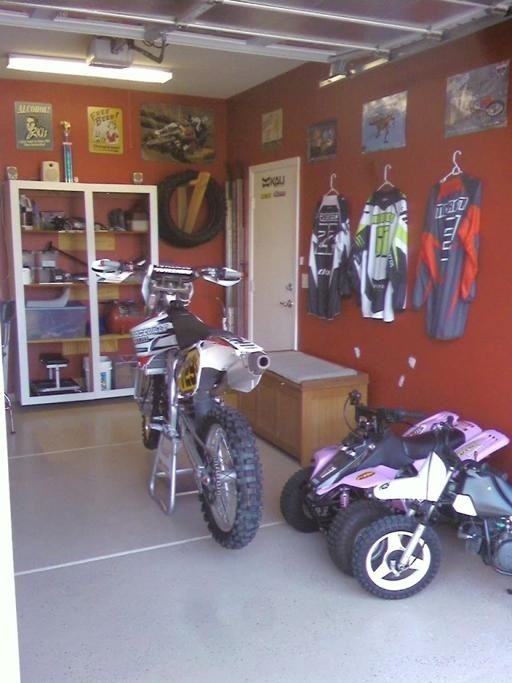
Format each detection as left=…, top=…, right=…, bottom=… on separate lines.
left=0, top=300, right=16, bottom=435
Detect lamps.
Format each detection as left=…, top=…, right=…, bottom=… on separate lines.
left=318, top=56, right=388, bottom=88
left=6, top=52, right=172, bottom=84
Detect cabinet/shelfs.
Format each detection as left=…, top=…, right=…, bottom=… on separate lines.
left=5, top=178, right=161, bottom=407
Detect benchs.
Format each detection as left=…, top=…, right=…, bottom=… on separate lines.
left=237, top=349, right=369, bottom=466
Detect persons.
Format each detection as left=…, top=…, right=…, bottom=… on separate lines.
left=25, top=116, right=48, bottom=140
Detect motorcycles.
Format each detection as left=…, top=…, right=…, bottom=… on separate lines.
left=348, top=418, right=511, bottom=600
left=91, top=258, right=270, bottom=552
left=143, top=114, right=209, bottom=155
left=279, top=387, right=510, bottom=579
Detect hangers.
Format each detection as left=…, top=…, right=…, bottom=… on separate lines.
left=440, top=151, right=462, bottom=183
left=324, top=173, right=339, bottom=196
left=376, top=164, right=394, bottom=191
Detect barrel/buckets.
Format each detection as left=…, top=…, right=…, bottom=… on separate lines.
left=82, top=355, right=113, bottom=391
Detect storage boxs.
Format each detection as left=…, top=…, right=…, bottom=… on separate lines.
left=25, top=302, right=86, bottom=341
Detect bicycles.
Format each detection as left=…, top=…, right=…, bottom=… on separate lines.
left=456, top=83, right=505, bottom=122
